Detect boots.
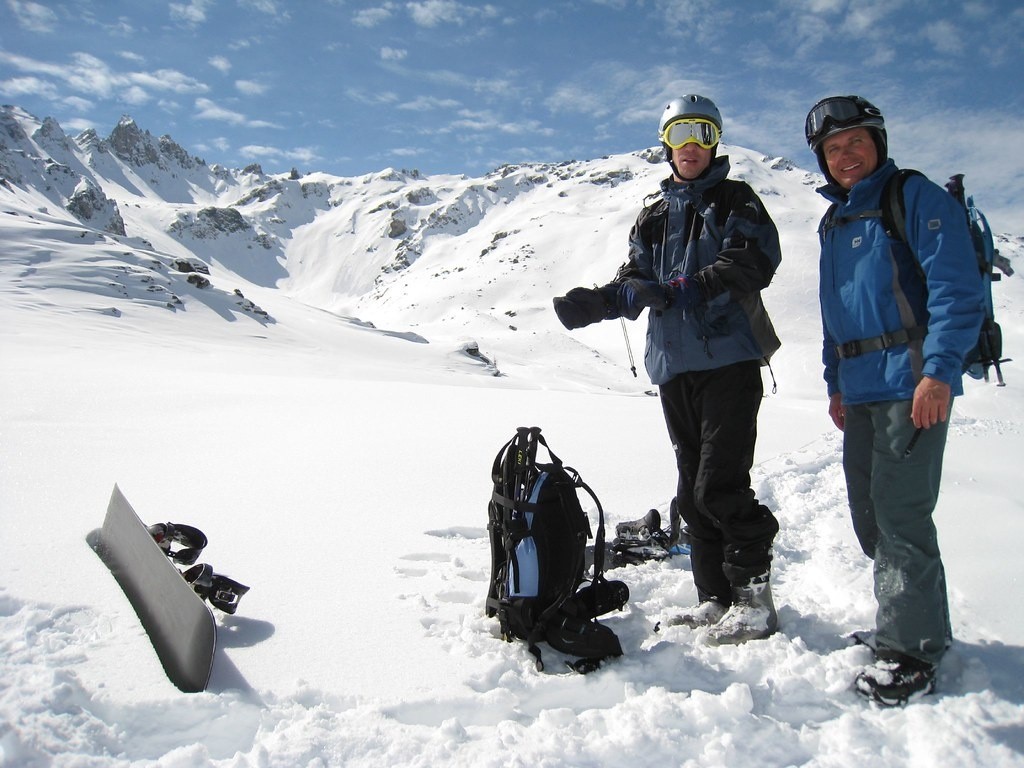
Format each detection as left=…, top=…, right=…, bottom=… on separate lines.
left=705, top=563, right=779, bottom=645
left=667, top=597, right=728, bottom=634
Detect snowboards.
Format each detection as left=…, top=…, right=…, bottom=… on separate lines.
left=83, top=480, right=220, bottom=695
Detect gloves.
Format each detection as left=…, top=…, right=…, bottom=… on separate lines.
left=553, top=287, right=608, bottom=331
left=615, top=279, right=671, bottom=321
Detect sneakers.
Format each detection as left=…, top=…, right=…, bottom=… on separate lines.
left=852, top=629, right=951, bottom=655
left=853, top=658, right=937, bottom=707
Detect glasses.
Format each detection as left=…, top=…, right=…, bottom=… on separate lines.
left=663, top=119, right=719, bottom=148
left=804, top=96, right=863, bottom=142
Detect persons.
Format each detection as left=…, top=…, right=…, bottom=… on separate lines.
left=552, top=94, right=782, bottom=647
left=802, top=95, right=989, bottom=708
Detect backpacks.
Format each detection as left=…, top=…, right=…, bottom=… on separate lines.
left=819, top=169, right=1014, bottom=387
left=507, top=464, right=605, bottom=669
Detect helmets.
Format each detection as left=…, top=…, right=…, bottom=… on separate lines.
left=810, top=95, right=887, bottom=186
left=658, top=94, right=723, bottom=144
